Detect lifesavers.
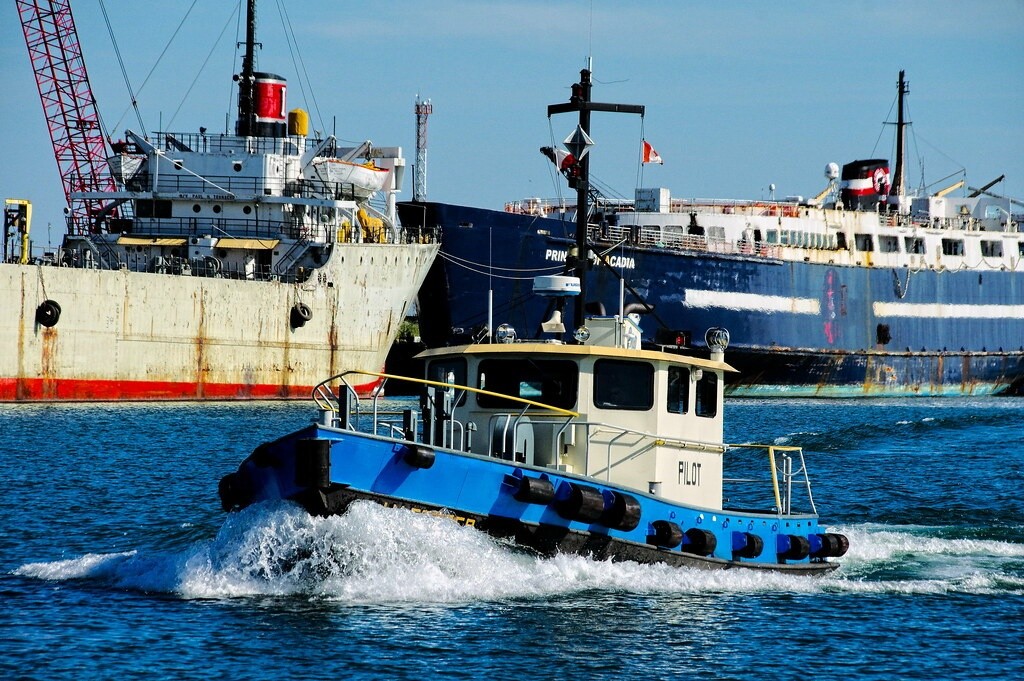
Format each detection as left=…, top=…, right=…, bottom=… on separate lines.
left=36, top=299, right=61, bottom=328
left=291, top=301, right=314, bottom=328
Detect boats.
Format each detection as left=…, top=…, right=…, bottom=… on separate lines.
left=216, top=55, right=850, bottom=587
left=393, top=70, right=1024, bottom=411
left=1, top=1, right=444, bottom=414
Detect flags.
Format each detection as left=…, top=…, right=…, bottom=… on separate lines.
left=554, top=145, right=581, bottom=173
left=642, top=138, right=664, bottom=165
